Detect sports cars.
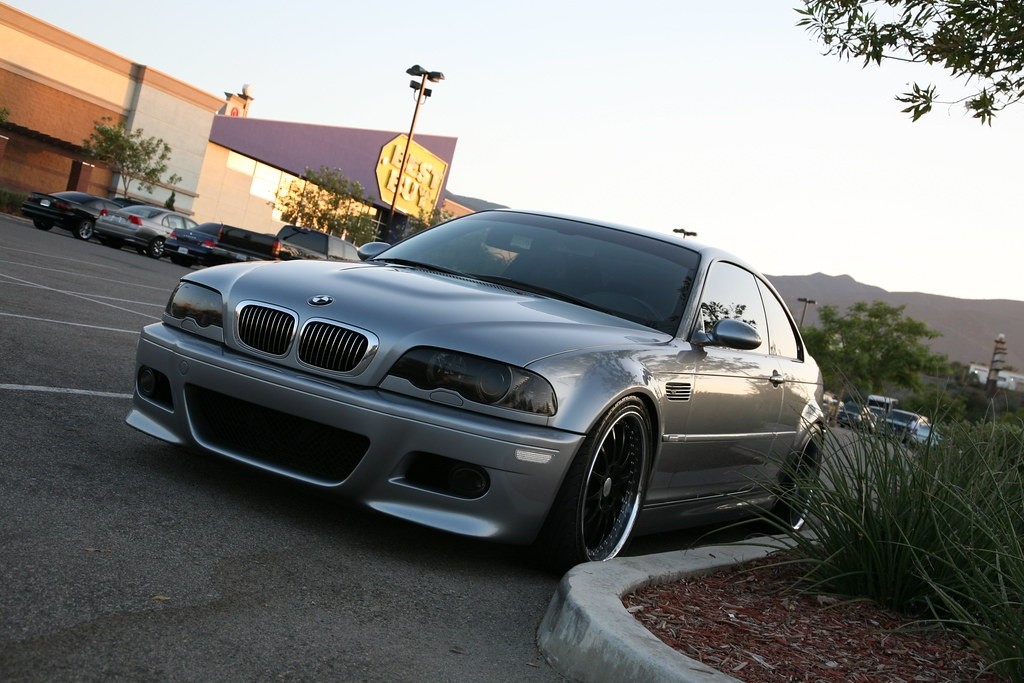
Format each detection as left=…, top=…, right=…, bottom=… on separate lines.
left=124, top=207, right=830, bottom=569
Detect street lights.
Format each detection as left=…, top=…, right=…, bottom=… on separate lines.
left=384, top=64, right=444, bottom=235
left=796, top=296, right=818, bottom=333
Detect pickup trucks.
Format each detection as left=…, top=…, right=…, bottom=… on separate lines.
left=194, top=225, right=362, bottom=270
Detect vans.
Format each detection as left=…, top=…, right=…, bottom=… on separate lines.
left=876, top=409, right=930, bottom=441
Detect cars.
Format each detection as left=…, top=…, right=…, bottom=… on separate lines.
left=908, top=422, right=941, bottom=448
left=19, top=190, right=126, bottom=248
left=163, top=222, right=220, bottom=266
left=837, top=402, right=872, bottom=429
left=92, top=205, right=202, bottom=259
left=822, top=394, right=839, bottom=422
